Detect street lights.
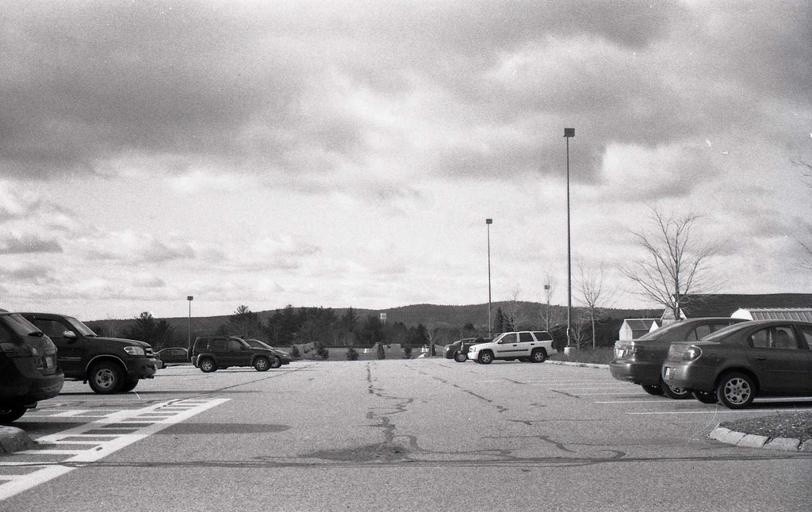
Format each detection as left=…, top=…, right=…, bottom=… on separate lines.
left=487, top=219, right=494, bottom=342
left=563, top=126, right=574, bottom=348
left=188, top=296, right=193, bottom=348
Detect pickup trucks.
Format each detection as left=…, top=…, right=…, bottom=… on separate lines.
left=460, top=333, right=501, bottom=355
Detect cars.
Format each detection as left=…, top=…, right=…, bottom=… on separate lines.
left=0, top=309, right=66, bottom=426
left=443, top=337, right=492, bottom=362
left=155, top=346, right=190, bottom=364
left=243, top=339, right=293, bottom=369
left=608, top=317, right=747, bottom=398
left=660, top=320, right=812, bottom=411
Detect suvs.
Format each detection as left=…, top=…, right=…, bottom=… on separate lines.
left=467, top=330, right=559, bottom=364
left=187, top=334, right=276, bottom=373
left=16, top=311, right=156, bottom=395
